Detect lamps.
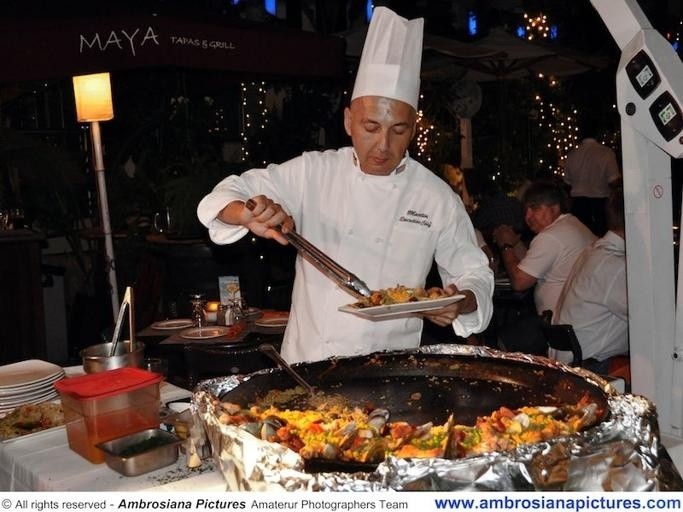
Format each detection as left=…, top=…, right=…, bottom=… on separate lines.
left=72, top=72, right=118, bottom=327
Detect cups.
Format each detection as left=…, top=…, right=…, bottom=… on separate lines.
left=142, top=358, right=161, bottom=374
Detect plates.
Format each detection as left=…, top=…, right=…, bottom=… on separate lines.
left=0, top=358, right=65, bottom=412
left=179, top=325, right=229, bottom=339
left=0, top=404, right=84, bottom=444
left=337, top=293, right=466, bottom=322
left=255, top=317, right=288, bottom=327
left=495, top=279, right=510, bottom=285
left=151, top=318, right=193, bottom=329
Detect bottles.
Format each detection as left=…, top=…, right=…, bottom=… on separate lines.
left=190, top=291, right=207, bottom=326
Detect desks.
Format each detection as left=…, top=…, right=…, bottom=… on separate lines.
left=84, top=225, right=533, bottom=379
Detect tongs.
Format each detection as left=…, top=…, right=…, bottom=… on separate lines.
left=109, top=286, right=137, bottom=356
left=245, top=199, right=371, bottom=302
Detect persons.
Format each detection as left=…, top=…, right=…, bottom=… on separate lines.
left=468, top=113, right=631, bottom=394
left=196, top=96, right=496, bottom=367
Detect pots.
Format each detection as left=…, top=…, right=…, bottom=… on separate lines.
left=221, top=352, right=611, bottom=473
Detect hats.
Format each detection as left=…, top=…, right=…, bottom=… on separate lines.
left=351, top=7, right=424, bottom=111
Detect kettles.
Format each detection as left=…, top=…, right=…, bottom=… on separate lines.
left=216, top=305, right=232, bottom=326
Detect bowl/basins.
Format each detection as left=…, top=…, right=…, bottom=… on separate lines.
left=79, top=340, right=145, bottom=373
